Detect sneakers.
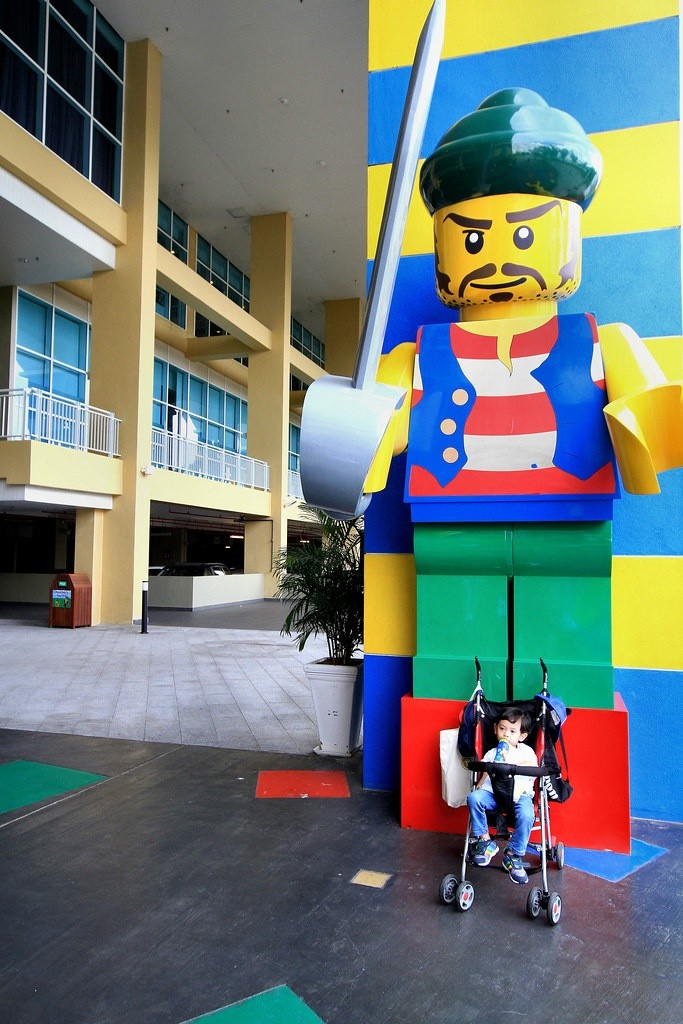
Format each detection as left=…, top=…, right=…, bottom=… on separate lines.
left=472, top=838, right=499, bottom=866
left=502, top=848, right=531, bottom=884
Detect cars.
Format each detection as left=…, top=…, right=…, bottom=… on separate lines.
left=156, top=562, right=232, bottom=576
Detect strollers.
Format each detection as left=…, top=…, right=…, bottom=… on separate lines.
left=438, top=655, right=566, bottom=925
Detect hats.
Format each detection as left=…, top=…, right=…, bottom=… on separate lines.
left=534, top=691, right=567, bottom=741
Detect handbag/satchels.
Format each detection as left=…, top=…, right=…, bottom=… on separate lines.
left=539, top=729, right=573, bottom=803
left=439, top=726, right=472, bottom=807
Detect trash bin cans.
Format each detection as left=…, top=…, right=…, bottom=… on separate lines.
left=50, top=574, right=91, bottom=629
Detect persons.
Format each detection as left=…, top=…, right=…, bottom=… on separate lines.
left=298, top=89, right=683, bottom=855
left=467, top=709, right=540, bottom=884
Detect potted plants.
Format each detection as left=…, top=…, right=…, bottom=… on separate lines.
left=270, top=503, right=364, bottom=758
